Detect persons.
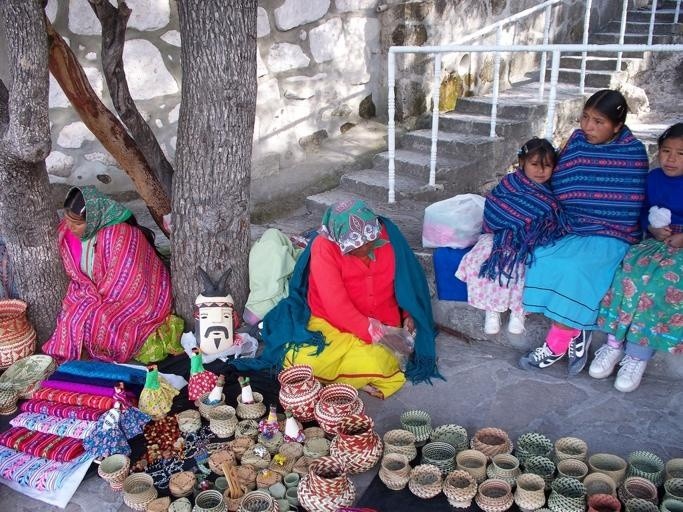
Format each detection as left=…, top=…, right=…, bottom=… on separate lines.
left=264, top=195, right=446, bottom=401
left=41, top=182, right=174, bottom=366
left=514, top=88, right=646, bottom=378
left=453, top=135, right=558, bottom=335
left=585, top=120, right=683, bottom=394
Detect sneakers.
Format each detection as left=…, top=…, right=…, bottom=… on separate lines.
left=508, top=311, right=527, bottom=334
left=519, top=341, right=565, bottom=371
left=589, top=342, right=625, bottom=379
left=485, top=311, right=502, bottom=334
left=613, top=353, right=648, bottom=392
left=567, top=330, right=593, bottom=375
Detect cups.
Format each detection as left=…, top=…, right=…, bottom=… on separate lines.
left=285, top=487, right=298, bottom=505
left=283, top=472, right=299, bottom=487
